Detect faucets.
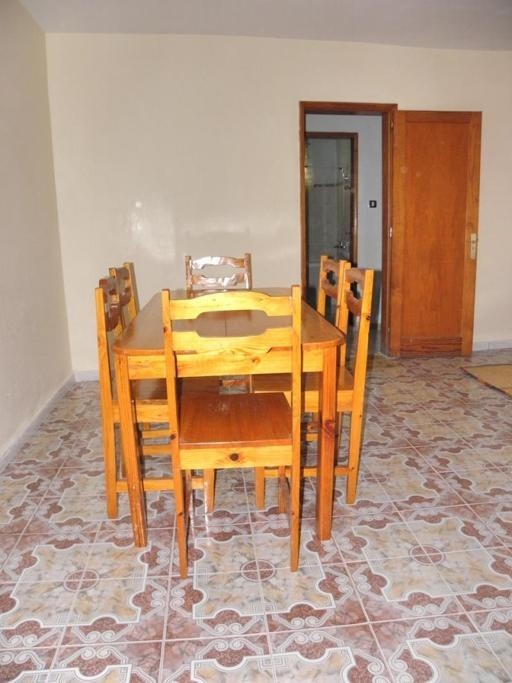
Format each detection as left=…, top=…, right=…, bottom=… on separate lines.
left=335, top=240, right=346, bottom=250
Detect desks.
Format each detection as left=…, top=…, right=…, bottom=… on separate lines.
left=111, top=285, right=348, bottom=548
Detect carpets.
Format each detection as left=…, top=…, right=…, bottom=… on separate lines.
left=457, top=362, right=512, bottom=401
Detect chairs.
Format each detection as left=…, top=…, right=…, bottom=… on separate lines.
left=158, top=281, right=304, bottom=576
left=249, top=253, right=354, bottom=374
left=182, top=252, right=254, bottom=291
left=249, top=261, right=375, bottom=508
left=107, top=260, right=178, bottom=428
left=94, top=275, right=216, bottom=524
